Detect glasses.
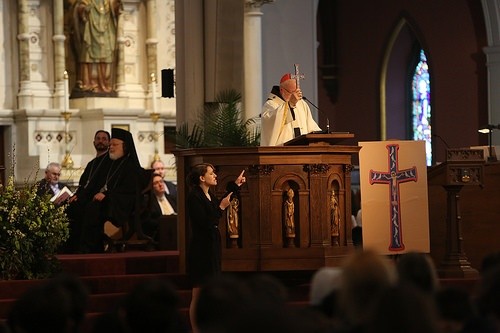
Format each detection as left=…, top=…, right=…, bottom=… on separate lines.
left=106, top=142, right=123, bottom=150
left=282, top=87, right=295, bottom=93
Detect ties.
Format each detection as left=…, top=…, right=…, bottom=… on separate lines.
left=160, top=198, right=170, bottom=216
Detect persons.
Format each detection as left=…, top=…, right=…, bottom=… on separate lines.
left=331, top=190, right=338, bottom=234
left=352, top=209, right=362, bottom=246
left=261, top=72, right=322, bottom=146
left=227, top=197, right=239, bottom=235
left=185, top=163, right=246, bottom=333
left=65, top=128, right=177, bottom=254
left=0, top=248, right=500, bottom=333
left=31, top=163, right=71, bottom=199
left=68, top=0, right=124, bottom=93
left=284, top=189, right=294, bottom=234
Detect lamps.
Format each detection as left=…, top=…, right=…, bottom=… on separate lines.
left=162, top=69, right=176, bottom=98
left=478, top=125, right=500, bottom=134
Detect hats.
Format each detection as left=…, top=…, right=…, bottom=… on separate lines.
left=310, top=268, right=340, bottom=306
left=280, top=73, right=291, bottom=83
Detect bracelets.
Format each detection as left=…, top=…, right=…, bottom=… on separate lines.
left=219, top=206, right=225, bottom=211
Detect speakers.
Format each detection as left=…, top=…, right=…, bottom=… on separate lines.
left=161, top=69, right=175, bottom=98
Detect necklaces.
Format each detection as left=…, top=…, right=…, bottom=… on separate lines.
left=100, top=156, right=124, bottom=192
left=85, top=153, right=107, bottom=189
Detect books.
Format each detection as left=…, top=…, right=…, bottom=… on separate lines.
left=50, top=186, right=72, bottom=208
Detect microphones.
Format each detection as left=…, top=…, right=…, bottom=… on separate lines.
left=302, top=97, right=330, bottom=134
left=431, top=135, right=451, bottom=148
